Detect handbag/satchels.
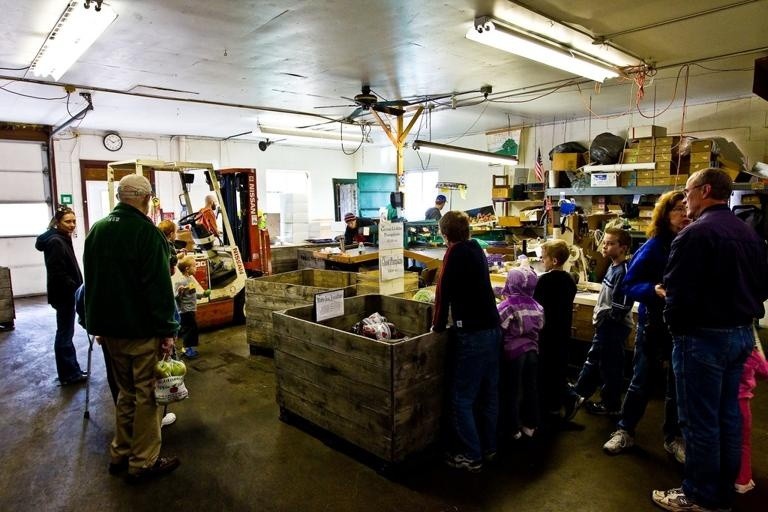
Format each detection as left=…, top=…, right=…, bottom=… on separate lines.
left=154, top=351, right=189, bottom=407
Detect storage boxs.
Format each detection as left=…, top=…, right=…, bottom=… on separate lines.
left=273, top=294, right=449, bottom=479
left=245, top=269, right=356, bottom=359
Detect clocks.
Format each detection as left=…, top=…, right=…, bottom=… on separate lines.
left=103, top=133, right=123, bottom=151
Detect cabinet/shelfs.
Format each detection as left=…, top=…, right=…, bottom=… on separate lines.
left=546, top=185, right=715, bottom=240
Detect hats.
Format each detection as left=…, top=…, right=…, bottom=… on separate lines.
left=344, top=213, right=355, bottom=222
left=436, top=195, right=448, bottom=203
left=55, top=202, right=75, bottom=219
left=117, top=175, right=154, bottom=195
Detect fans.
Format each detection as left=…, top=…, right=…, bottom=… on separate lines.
left=315, top=85, right=408, bottom=121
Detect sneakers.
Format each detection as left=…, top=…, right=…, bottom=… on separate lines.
left=80, top=371, right=91, bottom=375
left=585, top=399, right=622, bottom=416
left=185, top=353, right=199, bottom=359
left=549, top=407, right=560, bottom=414
left=479, top=448, right=496, bottom=461
left=442, top=450, right=482, bottom=474
left=62, top=374, right=89, bottom=386
left=158, top=413, right=176, bottom=427
left=180, top=351, right=187, bottom=357
left=514, top=431, right=522, bottom=438
left=518, top=420, right=534, bottom=436
left=601, top=429, right=635, bottom=455
left=652, top=487, right=709, bottom=512
left=562, top=396, right=584, bottom=418
left=734, top=479, right=755, bottom=494
left=133, top=455, right=179, bottom=485
left=664, top=436, right=687, bottom=464
left=109, top=459, right=130, bottom=474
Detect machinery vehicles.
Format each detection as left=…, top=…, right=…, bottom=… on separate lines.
left=107, top=158, right=273, bottom=328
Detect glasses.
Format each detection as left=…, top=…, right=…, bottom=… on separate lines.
left=682, top=182, right=709, bottom=192
left=670, top=205, right=687, bottom=216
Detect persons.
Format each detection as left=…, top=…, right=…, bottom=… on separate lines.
left=425, top=195, right=448, bottom=220
left=173, top=256, right=208, bottom=359
left=36, top=202, right=88, bottom=386
left=734, top=349, right=768, bottom=494
left=429, top=211, right=503, bottom=474
left=196, top=196, right=223, bottom=236
left=85, top=174, right=183, bottom=478
left=344, top=213, right=378, bottom=246
left=533, top=239, right=586, bottom=421
left=75, top=246, right=181, bottom=429
left=602, top=191, right=694, bottom=463
left=651, top=169, right=767, bottom=512
left=496, top=268, right=545, bottom=439
left=567, top=227, right=634, bottom=413
left=158, top=220, right=187, bottom=258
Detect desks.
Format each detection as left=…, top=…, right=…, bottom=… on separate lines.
left=319, top=245, right=380, bottom=271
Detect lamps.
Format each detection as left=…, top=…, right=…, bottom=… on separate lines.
left=413, top=139, right=519, bottom=171
